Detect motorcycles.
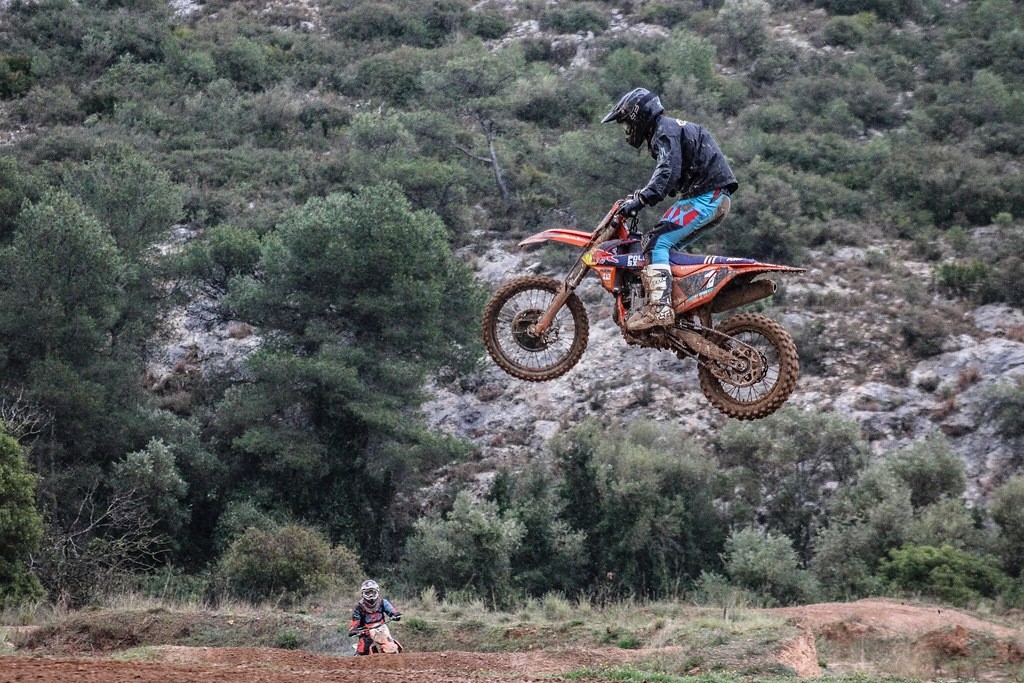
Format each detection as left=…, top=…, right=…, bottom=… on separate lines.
left=480, top=194, right=808, bottom=421
left=349, top=615, right=404, bottom=655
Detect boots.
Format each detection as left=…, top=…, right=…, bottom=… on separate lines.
left=626, top=264, right=676, bottom=330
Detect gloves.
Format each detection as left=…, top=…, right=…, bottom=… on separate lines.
left=615, top=198, right=646, bottom=219
left=391, top=612, right=402, bottom=621
left=349, top=628, right=359, bottom=637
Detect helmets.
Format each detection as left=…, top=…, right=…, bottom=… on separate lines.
left=600, top=87, right=664, bottom=149
left=361, top=580, right=380, bottom=605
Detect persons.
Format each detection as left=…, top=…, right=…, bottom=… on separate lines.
left=599, top=87, right=740, bottom=330
left=350, top=580, right=402, bottom=655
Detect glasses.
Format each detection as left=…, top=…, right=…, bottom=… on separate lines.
left=617, top=115, right=632, bottom=134
